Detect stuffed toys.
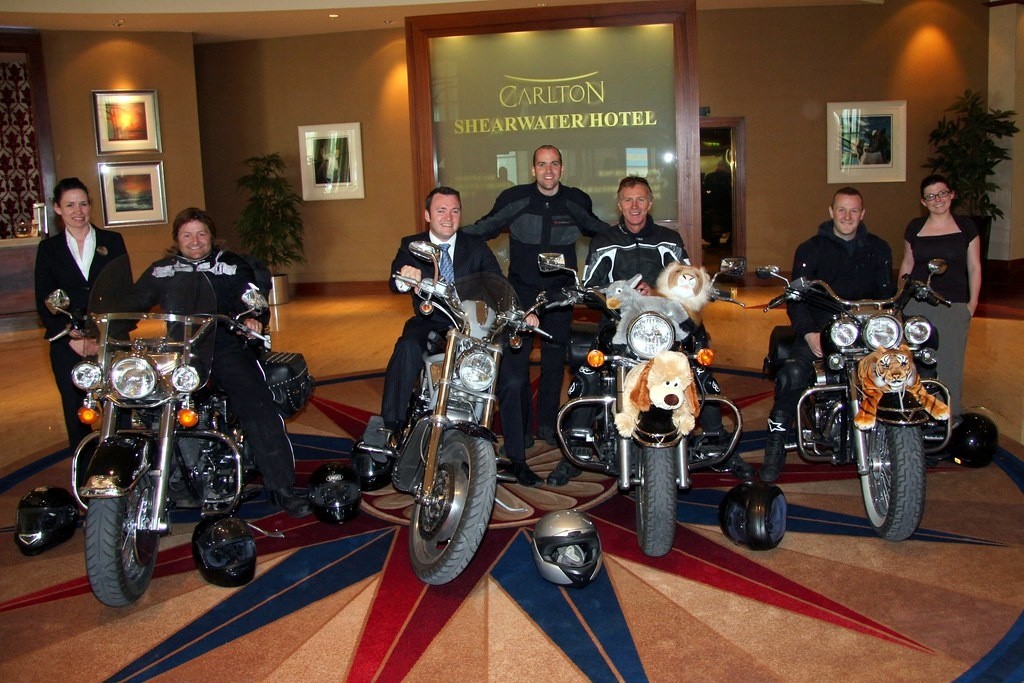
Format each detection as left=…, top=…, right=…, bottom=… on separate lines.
left=600, top=261, right=713, bottom=347
left=615, top=350, right=701, bottom=436
left=854, top=344, right=951, bottom=431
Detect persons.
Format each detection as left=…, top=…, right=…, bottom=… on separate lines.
left=381, top=187, right=544, bottom=488
left=758, top=187, right=940, bottom=482
left=547, top=175, right=758, bottom=487
left=897, top=175, right=981, bottom=426
left=67, top=208, right=314, bottom=519
left=34, top=178, right=133, bottom=475
left=458, top=145, right=610, bottom=454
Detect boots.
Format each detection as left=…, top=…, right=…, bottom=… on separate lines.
left=759, top=411, right=793, bottom=480
left=707, top=437, right=756, bottom=480
left=547, top=457, right=583, bottom=486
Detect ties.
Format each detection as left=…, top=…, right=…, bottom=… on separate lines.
left=436, top=243, right=455, bottom=289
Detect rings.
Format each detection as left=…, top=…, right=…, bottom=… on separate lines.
left=256, top=326, right=260, bottom=331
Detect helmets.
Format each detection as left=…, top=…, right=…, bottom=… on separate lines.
left=15, top=486, right=78, bottom=556
left=531, top=508, right=603, bottom=589
left=348, top=440, right=396, bottom=492
left=191, top=513, right=257, bottom=588
left=307, top=462, right=362, bottom=524
left=946, top=412, right=998, bottom=467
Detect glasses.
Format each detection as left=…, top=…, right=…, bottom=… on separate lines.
left=923, top=190, right=951, bottom=201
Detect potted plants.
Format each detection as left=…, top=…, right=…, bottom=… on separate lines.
left=918, top=87, right=1021, bottom=277
left=230, top=151, right=310, bottom=305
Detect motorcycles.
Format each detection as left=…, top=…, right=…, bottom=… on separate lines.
left=356, top=242, right=554, bottom=585
left=46, top=249, right=317, bottom=608
left=756, top=257, right=953, bottom=541
left=539, top=252, right=746, bottom=555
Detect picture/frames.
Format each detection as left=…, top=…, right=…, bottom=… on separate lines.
left=91, top=89, right=163, bottom=157
left=98, top=160, right=170, bottom=229
left=826, top=100, right=906, bottom=183
left=298, top=122, right=365, bottom=201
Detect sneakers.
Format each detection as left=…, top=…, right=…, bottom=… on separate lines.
left=507, top=461, right=545, bottom=487
left=718, top=481, right=788, bottom=551
left=269, top=488, right=313, bottom=518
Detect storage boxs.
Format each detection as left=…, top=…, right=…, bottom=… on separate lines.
left=259, top=350, right=315, bottom=417
left=566, top=320, right=597, bottom=376
left=762, top=323, right=794, bottom=382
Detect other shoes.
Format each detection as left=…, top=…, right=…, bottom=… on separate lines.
left=498, top=435, right=535, bottom=455
left=537, top=432, right=561, bottom=444
